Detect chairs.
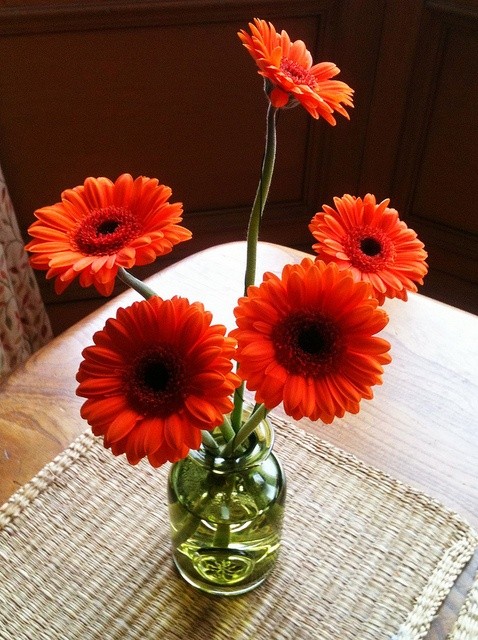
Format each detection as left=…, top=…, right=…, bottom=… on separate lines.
left=0, top=175, right=54, bottom=393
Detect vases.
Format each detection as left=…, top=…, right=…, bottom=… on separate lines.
left=167, top=407, right=289, bottom=599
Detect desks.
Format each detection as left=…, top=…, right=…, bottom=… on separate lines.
left=0, top=236, right=478, bottom=640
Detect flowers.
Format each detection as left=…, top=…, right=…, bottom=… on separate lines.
left=20, top=14, right=431, bottom=553
